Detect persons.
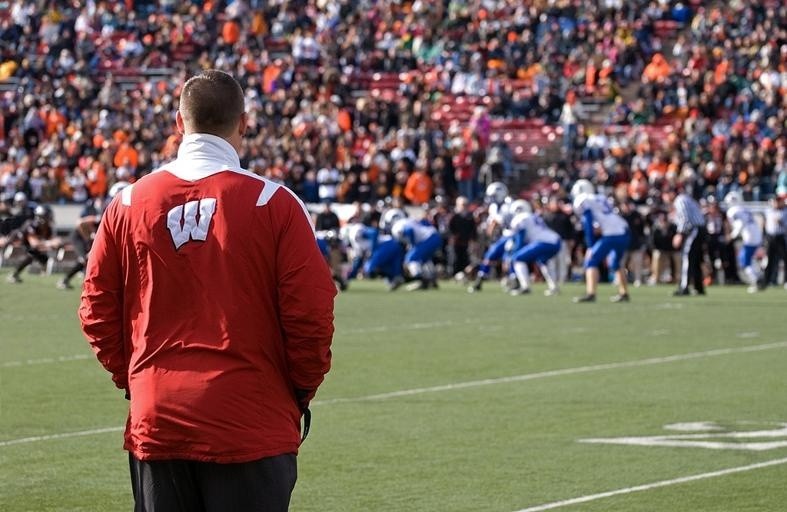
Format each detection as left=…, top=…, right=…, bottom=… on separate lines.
left=2, top=0, right=787, bottom=305
left=77, top=70, right=337, bottom=512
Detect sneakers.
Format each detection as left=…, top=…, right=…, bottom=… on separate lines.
left=454, top=271, right=561, bottom=296
left=573, top=295, right=595, bottom=302
left=386, top=277, right=438, bottom=289
left=669, top=287, right=704, bottom=296
left=610, top=292, right=629, bottom=302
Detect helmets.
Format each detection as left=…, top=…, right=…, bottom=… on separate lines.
left=34, top=206, right=52, bottom=224
left=724, top=190, right=743, bottom=208
left=338, top=224, right=351, bottom=246
left=571, top=178, right=596, bottom=199
left=384, top=208, right=406, bottom=232
left=509, top=199, right=532, bottom=217
left=486, top=182, right=508, bottom=204
